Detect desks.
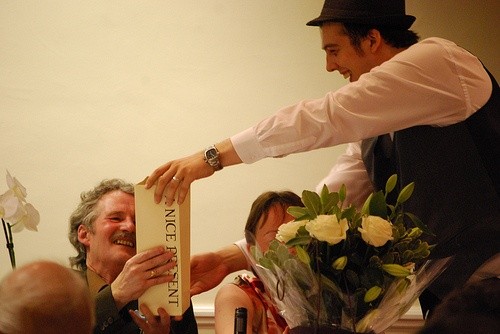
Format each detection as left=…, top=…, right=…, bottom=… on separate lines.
left=192, top=295, right=427, bottom=334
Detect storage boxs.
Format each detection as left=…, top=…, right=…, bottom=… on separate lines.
left=133, top=175, right=192, bottom=317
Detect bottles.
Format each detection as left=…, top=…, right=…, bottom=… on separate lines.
left=234, top=307, right=247, bottom=334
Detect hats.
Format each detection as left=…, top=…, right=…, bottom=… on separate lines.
left=306, top=0, right=416, bottom=31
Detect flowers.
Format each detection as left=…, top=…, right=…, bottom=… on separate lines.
left=232, top=173, right=454, bottom=334
left=0, top=169, right=41, bottom=270
